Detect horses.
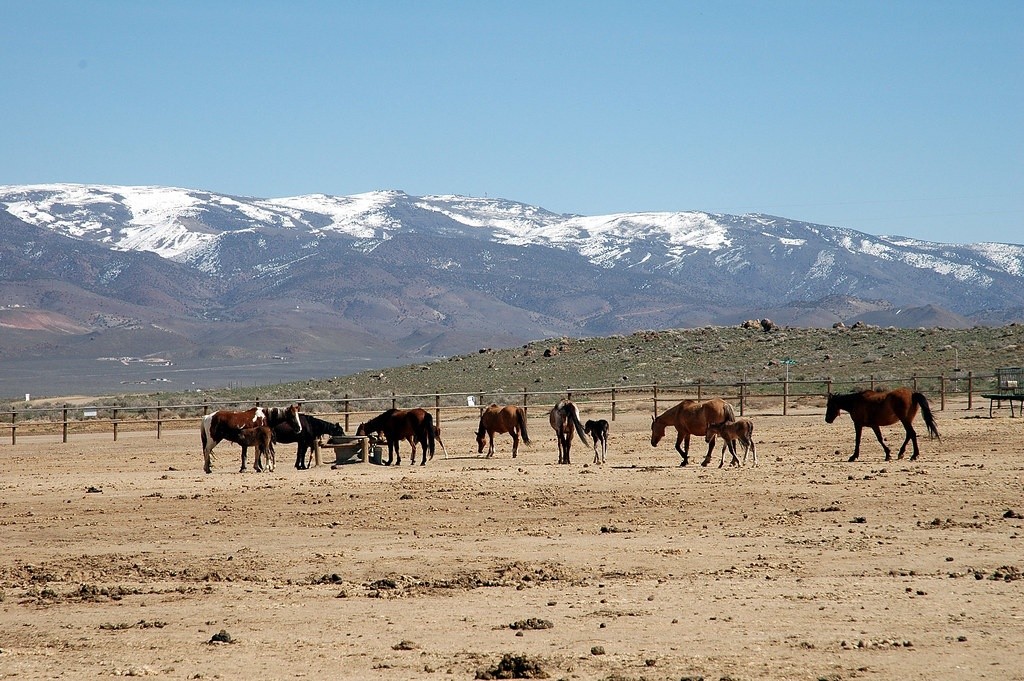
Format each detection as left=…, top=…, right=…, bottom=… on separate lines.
left=584, top=419, right=609, bottom=464
left=233, top=413, right=347, bottom=474
left=200, top=403, right=302, bottom=473
left=705, top=419, right=757, bottom=468
left=547, top=399, right=592, bottom=464
left=474, top=404, right=532, bottom=459
left=356, top=408, right=449, bottom=466
left=825, top=387, right=942, bottom=462
left=651, top=398, right=737, bottom=468
